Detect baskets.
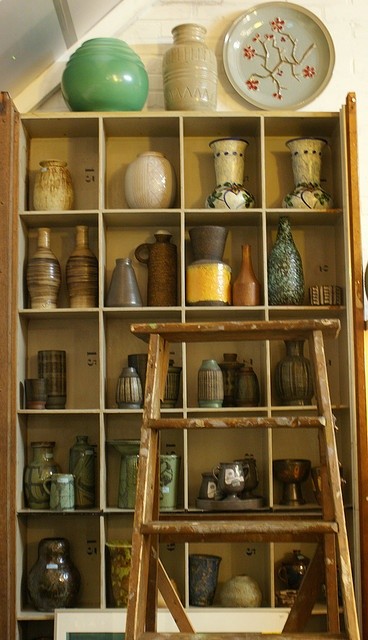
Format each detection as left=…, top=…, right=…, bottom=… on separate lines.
left=309, top=285, right=342, bottom=305
left=275, top=590, right=298, bottom=607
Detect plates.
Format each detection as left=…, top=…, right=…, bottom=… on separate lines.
left=222, top=1, right=337, bottom=112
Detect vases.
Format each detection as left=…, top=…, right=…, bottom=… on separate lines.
left=22, top=441, right=63, bottom=510
left=159, top=359, right=182, bottom=408
left=234, top=365, right=260, bottom=407
left=185, top=225, right=232, bottom=306
left=127, top=353, right=155, bottom=410
left=59, top=37, right=149, bottom=112
left=187, top=552, right=223, bottom=605
left=68, top=434, right=98, bottom=509
left=27, top=537, right=81, bottom=612
left=214, top=350, right=244, bottom=407
left=230, top=244, right=261, bottom=307
left=35, top=348, right=68, bottom=410
left=267, top=215, right=306, bottom=305
left=114, top=366, right=145, bottom=409
left=219, top=573, right=262, bottom=610
left=26, top=227, right=62, bottom=310
left=281, top=135, right=335, bottom=208
left=158, top=453, right=180, bottom=512
left=22, top=378, right=46, bottom=408
left=32, top=157, right=75, bottom=212
left=66, top=224, right=98, bottom=308
left=277, top=548, right=314, bottom=587
left=162, top=23, right=219, bottom=111
left=146, top=229, right=179, bottom=307
left=274, top=335, right=314, bottom=406
left=207, top=137, right=256, bottom=211
left=106, top=438, right=142, bottom=509
left=122, top=150, right=178, bottom=209
left=196, top=357, right=225, bottom=408
left=105, top=257, right=144, bottom=308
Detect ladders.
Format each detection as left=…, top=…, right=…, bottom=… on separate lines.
left=125, top=318, right=361, bottom=640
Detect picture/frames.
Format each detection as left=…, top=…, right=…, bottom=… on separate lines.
left=53, top=605, right=292, bottom=640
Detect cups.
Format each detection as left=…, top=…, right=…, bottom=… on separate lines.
left=41, top=472, right=76, bottom=511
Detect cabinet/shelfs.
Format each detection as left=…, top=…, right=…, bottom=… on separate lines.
left=0, top=91, right=367, bottom=640
